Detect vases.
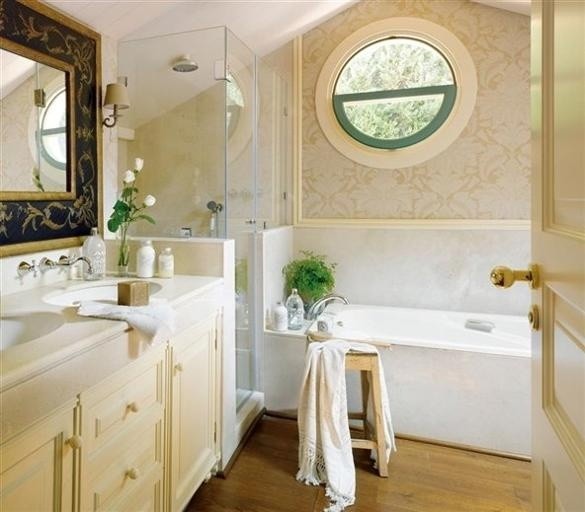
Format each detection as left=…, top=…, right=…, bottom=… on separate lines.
left=119, top=239, right=130, bottom=273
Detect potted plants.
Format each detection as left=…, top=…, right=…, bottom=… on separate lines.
left=282, top=248, right=339, bottom=319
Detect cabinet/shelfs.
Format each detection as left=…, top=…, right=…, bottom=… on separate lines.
left=0, top=285, right=223, bottom=512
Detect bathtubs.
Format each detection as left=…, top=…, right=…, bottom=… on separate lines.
left=306, top=302, right=531, bottom=358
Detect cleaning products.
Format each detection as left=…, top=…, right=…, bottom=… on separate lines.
left=287, top=289, right=304, bottom=330
left=82, top=228, right=105, bottom=276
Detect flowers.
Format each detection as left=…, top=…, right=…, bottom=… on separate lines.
left=107, top=158, right=157, bottom=265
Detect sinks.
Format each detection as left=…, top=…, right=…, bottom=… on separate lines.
left=44, top=279, right=163, bottom=306
left=0, top=310, right=66, bottom=350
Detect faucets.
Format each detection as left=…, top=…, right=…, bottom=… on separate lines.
left=41, top=257, right=94, bottom=274
left=308, top=295, right=349, bottom=320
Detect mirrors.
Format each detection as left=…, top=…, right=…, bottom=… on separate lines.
left=0, top=1, right=103, bottom=258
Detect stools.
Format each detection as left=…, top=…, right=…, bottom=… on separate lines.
left=347, top=355, right=388, bottom=477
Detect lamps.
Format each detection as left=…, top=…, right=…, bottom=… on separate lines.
left=104, top=84, right=131, bottom=129
